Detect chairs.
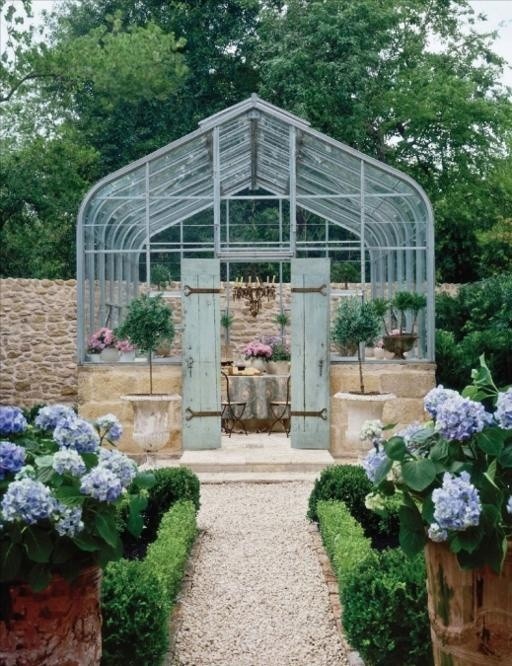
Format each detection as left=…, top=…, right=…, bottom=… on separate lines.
left=220, top=371, right=248, bottom=439
left=267, top=375, right=291, bottom=438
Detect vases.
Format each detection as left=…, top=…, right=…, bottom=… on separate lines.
left=86, top=353, right=100, bottom=362
left=253, top=356, right=266, bottom=370
left=100, top=346, right=119, bottom=362
left=423, top=538, right=511, bottom=666
left=0, top=567, right=102, bottom=666
left=266, top=360, right=289, bottom=376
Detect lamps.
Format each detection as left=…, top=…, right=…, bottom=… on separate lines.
left=231, top=176, right=276, bottom=319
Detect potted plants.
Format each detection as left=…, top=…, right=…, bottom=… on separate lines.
left=372, top=290, right=427, bottom=360
left=328, top=294, right=396, bottom=460
left=113, top=287, right=181, bottom=453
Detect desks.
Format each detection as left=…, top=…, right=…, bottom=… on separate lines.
left=221, top=375, right=291, bottom=433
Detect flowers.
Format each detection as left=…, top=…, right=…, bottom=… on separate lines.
left=239, top=340, right=273, bottom=360
left=359, top=354, right=511, bottom=573
left=0, top=400, right=156, bottom=592
left=87, top=327, right=133, bottom=353
left=261, top=335, right=290, bottom=361
